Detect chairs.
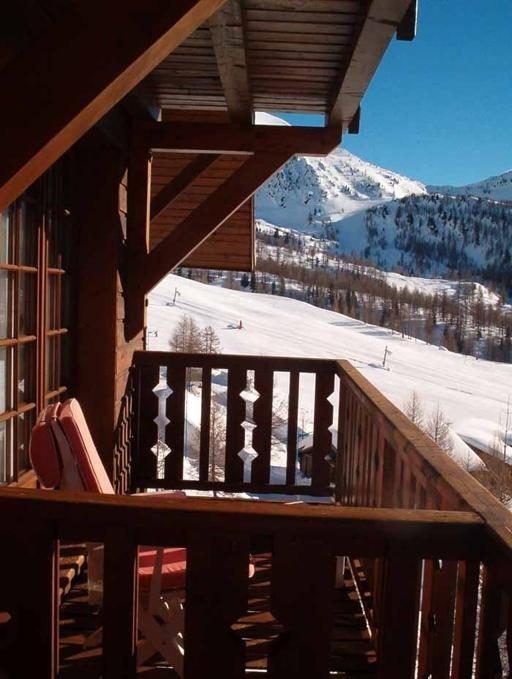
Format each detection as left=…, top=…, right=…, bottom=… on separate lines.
left=28, top=397, right=255, bottom=679
